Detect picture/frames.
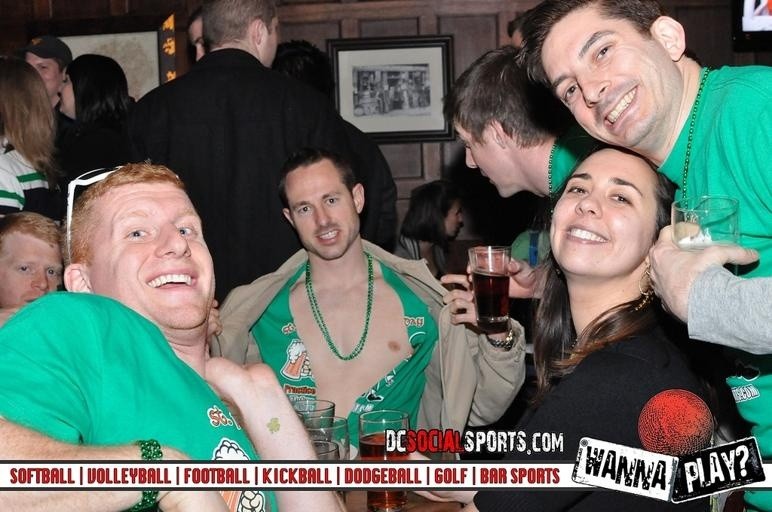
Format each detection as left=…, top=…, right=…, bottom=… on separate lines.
left=27, top=11, right=174, bottom=109
left=323, top=34, right=455, bottom=144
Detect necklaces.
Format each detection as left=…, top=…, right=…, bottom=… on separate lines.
left=567, top=296, right=648, bottom=353
left=304, top=250, right=375, bottom=361
left=547, top=145, right=558, bottom=213
left=680, top=62, right=716, bottom=218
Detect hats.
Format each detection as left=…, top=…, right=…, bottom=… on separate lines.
left=13, top=34, right=72, bottom=66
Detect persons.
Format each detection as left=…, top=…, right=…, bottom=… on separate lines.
left=0, top=1, right=464, bottom=332
left=404, top=144, right=772, bottom=512
left=0, top=163, right=345, bottom=512
left=514, top=0, right=771, bottom=354
left=440, top=43, right=590, bottom=297
left=207, top=146, right=526, bottom=461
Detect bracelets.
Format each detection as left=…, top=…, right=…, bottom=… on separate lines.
left=127, top=439, right=163, bottom=512
left=487, top=329, right=514, bottom=351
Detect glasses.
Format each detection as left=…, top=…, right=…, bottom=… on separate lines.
left=65, top=166, right=126, bottom=264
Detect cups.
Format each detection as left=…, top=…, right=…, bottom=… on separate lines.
left=288, top=396, right=410, bottom=512
left=670, top=194, right=741, bottom=276
left=469, top=244, right=511, bottom=335
